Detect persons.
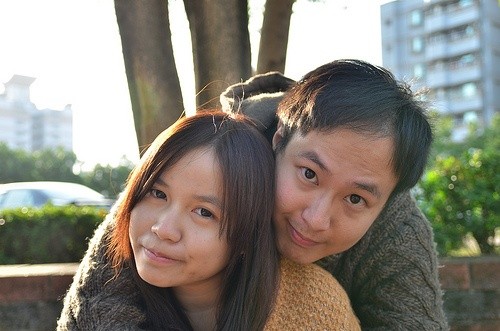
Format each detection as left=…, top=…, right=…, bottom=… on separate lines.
left=56, top=58, right=451, bottom=331
left=101, top=107, right=362, bottom=331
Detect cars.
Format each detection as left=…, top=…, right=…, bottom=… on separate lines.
left=0, top=181, right=118, bottom=215
